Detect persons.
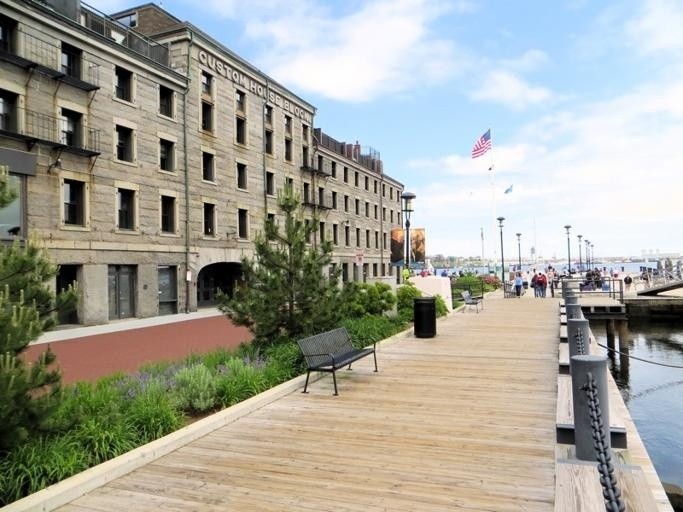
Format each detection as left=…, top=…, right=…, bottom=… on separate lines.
left=665, top=256, right=673, bottom=280
left=400, top=263, right=619, bottom=299
left=655, top=259, right=664, bottom=278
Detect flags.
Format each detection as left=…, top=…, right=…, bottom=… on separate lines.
left=470, top=129, right=491, bottom=160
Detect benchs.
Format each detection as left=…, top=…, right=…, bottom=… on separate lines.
left=460, top=289, right=483, bottom=313
left=296, top=327, right=378, bottom=395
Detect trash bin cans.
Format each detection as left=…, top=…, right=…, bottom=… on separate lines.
left=414, top=297, right=436, bottom=338
left=523, top=282, right=528, bottom=289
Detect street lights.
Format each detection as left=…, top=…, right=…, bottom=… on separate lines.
left=516, top=232, right=522, bottom=270
left=564, top=224, right=572, bottom=277
left=400, top=192, right=416, bottom=272
left=577, top=234, right=582, bottom=272
left=496, top=216, right=505, bottom=284
left=583, top=239, right=594, bottom=271
